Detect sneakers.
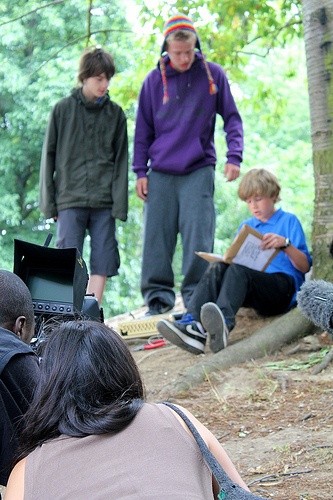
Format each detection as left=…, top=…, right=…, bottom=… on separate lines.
left=200, top=302, right=229, bottom=353
left=157, top=314, right=207, bottom=355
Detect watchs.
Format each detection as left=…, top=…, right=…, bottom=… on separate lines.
left=279, top=237, right=291, bottom=250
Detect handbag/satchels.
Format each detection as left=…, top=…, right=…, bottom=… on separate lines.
left=219, top=482, right=267, bottom=500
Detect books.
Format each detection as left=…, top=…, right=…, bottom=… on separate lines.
left=194, top=224, right=281, bottom=272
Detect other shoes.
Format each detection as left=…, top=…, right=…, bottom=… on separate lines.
left=145, top=302, right=162, bottom=315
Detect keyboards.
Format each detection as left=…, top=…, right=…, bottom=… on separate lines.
left=119, top=315, right=174, bottom=339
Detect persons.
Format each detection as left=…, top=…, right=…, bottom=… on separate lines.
left=4, top=321, right=251, bottom=500
left=156, top=168, right=313, bottom=355
left=39, top=48, right=128, bottom=305
left=132, top=15, right=244, bottom=315
left=0, top=270, right=41, bottom=500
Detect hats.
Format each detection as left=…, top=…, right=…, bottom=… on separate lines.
left=159, top=15, right=217, bottom=104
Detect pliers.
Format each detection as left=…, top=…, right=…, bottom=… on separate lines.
left=134, top=340, right=165, bottom=351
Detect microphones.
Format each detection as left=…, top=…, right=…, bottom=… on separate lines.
left=297, top=280, right=333, bottom=335
